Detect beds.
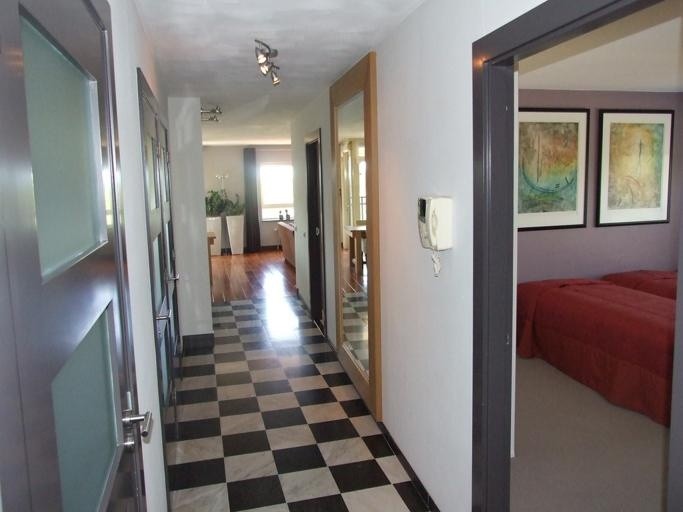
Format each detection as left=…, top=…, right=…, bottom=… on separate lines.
left=516, top=269, right=679, bottom=428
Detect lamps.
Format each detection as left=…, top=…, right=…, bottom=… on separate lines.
left=200, top=105, right=222, bottom=123
left=252, top=38, right=284, bottom=89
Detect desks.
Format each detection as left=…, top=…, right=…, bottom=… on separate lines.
left=344, top=225, right=367, bottom=276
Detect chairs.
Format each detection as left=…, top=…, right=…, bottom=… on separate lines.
left=276, top=222, right=295, bottom=268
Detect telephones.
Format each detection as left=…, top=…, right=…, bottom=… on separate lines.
left=418, top=197, right=453, bottom=251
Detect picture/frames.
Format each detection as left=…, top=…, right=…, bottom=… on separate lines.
left=517, top=107, right=675, bottom=233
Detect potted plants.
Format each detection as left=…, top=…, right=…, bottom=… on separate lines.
left=204, top=188, right=245, bottom=256
